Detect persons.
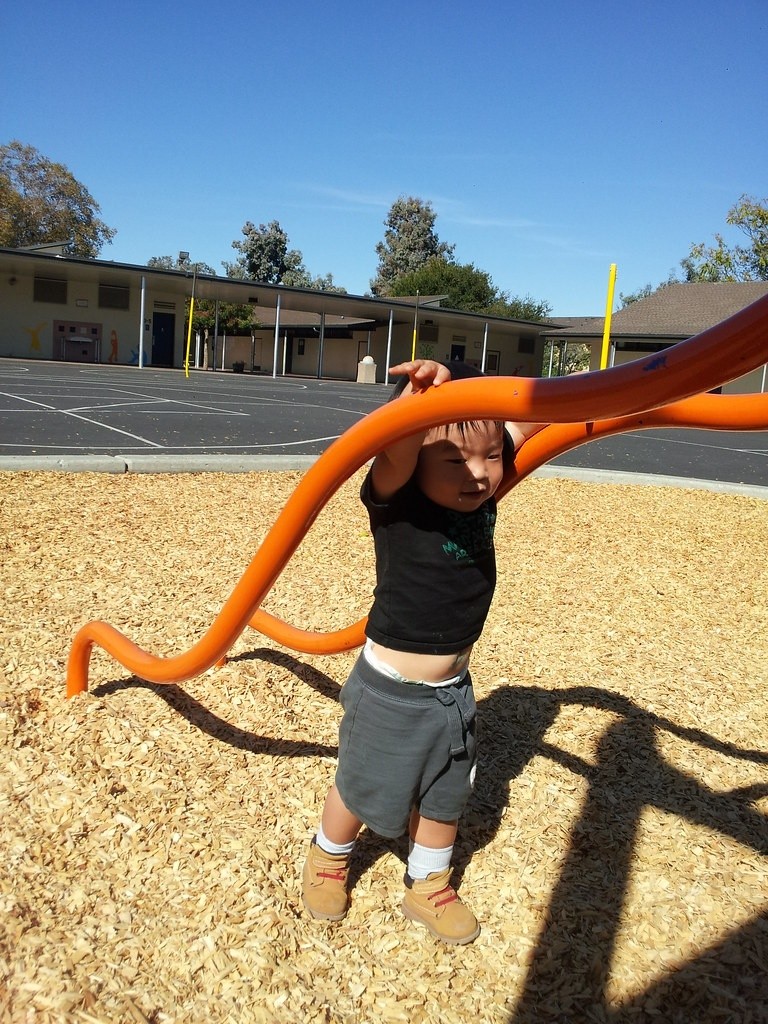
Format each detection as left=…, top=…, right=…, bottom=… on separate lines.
left=302, top=358, right=550, bottom=946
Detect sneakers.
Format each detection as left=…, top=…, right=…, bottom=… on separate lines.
left=302, top=833, right=350, bottom=921
left=401, top=866, right=481, bottom=945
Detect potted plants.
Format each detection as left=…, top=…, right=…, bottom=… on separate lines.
left=232, top=361, right=245, bottom=372
